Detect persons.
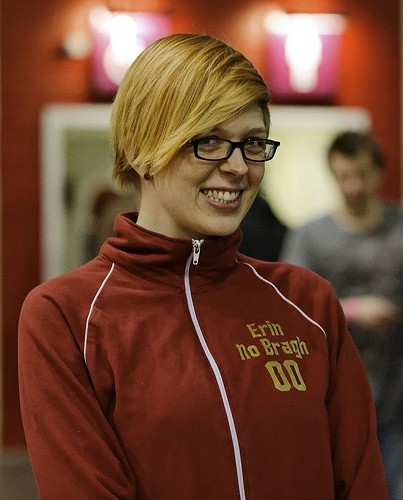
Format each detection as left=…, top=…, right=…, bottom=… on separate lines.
left=17, top=33, right=389, bottom=500
left=280, top=131, right=402, bottom=428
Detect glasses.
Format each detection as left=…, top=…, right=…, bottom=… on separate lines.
left=185, top=136, right=280, bottom=162
